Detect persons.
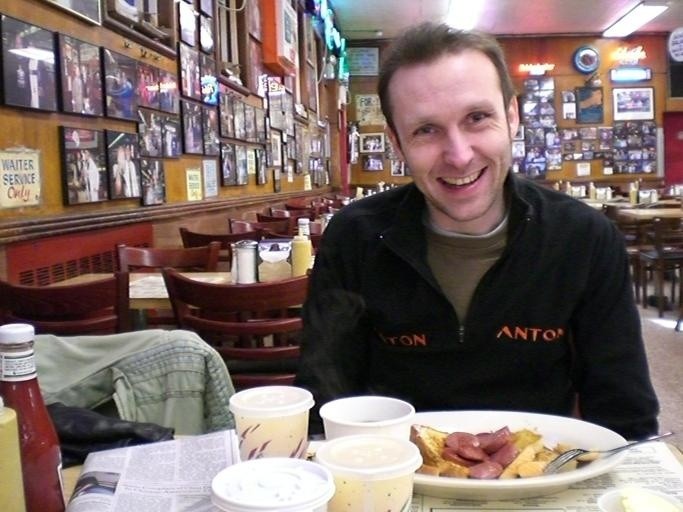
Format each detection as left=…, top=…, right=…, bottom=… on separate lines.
left=112, top=144, right=140, bottom=197
left=111, top=71, right=132, bottom=117
left=139, top=70, right=156, bottom=105
left=71, top=149, right=99, bottom=201
left=72, top=66, right=83, bottom=113
left=71, top=130, right=80, bottom=146
left=295, top=21, right=659, bottom=441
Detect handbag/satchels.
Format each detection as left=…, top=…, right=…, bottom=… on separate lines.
left=43, top=398, right=178, bottom=472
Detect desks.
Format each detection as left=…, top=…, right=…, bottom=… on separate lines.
left=61, top=416, right=683, bottom=511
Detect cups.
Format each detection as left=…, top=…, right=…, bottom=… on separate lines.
left=315, top=434, right=424, bottom=512
left=320, top=395, right=417, bottom=441
left=227, top=383, right=318, bottom=460
left=597, top=486, right=681, bottom=512
left=210, top=456, right=337, bottom=511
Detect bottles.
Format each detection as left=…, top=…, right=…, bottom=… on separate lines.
left=630, top=183, right=638, bottom=203
left=298, top=217, right=310, bottom=240
left=1, top=322, right=68, bottom=512
left=589, top=181, right=596, bottom=200
left=566, top=181, right=571, bottom=197
left=291, top=235, right=312, bottom=278
left=0, top=397, right=26, bottom=512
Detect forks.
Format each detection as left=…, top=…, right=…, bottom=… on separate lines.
left=540, top=430, right=676, bottom=475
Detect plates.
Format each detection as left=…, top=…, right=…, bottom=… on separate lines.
left=413, top=408, right=629, bottom=501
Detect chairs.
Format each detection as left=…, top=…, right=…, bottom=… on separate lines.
left=602, top=197, right=683, bottom=333
left=1, top=194, right=368, bottom=395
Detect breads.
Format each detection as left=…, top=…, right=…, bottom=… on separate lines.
left=409, top=424, right=543, bottom=479
